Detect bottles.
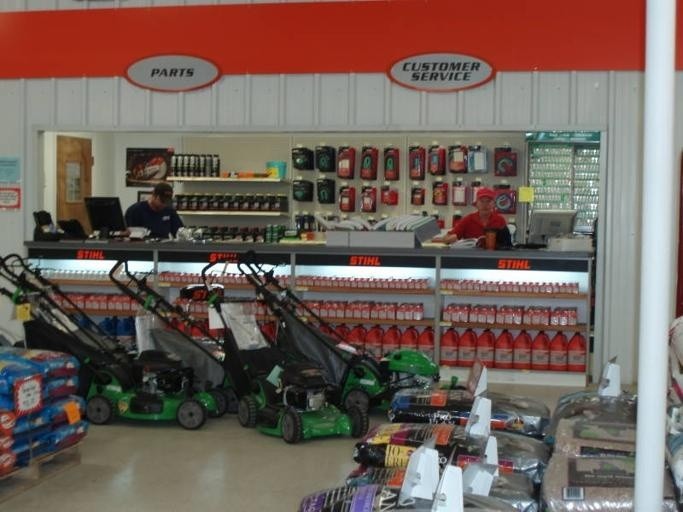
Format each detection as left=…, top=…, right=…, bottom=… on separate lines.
left=169, top=152, right=219, bottom=178
left=168, top=193, right=335, bottom=244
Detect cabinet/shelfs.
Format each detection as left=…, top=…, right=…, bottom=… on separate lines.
left=24, top=239, right=594, bottom=387
left=166, top=176, right=289, bottom=217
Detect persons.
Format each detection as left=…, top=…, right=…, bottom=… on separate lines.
left=124, top=181, right=185, bottom=242
left=440, top=187, right=510, bottom=250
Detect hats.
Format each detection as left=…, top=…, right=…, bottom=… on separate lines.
left=154, top=183, right=173, bottom=204
left=476, top=188, right=496, bottom=201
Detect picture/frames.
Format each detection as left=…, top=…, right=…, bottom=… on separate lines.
left=138, top=191, right=153, bottom=202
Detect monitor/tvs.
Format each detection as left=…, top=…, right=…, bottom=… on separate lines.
left=528, top=208, right=578, bottom=246
left=85, top=197, right=126, bottom=240
